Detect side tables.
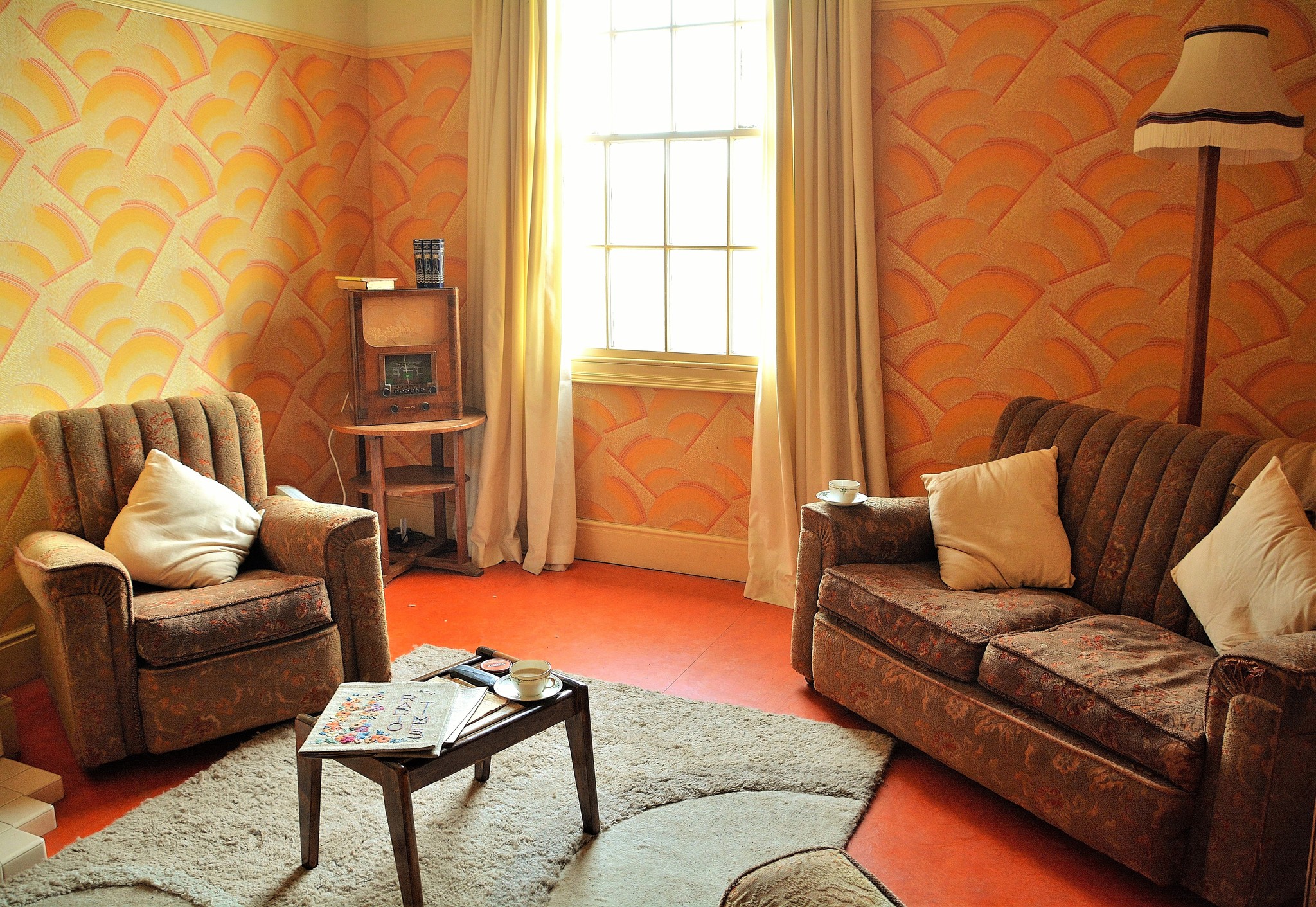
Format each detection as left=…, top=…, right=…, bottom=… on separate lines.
left=328, top=402, right=488, bottom=589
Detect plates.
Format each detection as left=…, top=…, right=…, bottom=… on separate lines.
left=493, top=674, right=562, bottom=701
left=816, top=490, right=868, bottom=506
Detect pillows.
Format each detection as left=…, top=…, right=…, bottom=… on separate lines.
left=919, top=445, right=1076, bottom=592
left=102, top=446, right=265, bottom=589
left=1170, top=454, right=1316, bottom=653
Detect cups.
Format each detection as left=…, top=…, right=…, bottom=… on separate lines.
left=829, top=479, right=861, bottom=502
left=509, top=659, right=555, bottom=697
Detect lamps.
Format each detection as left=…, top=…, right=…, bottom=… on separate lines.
left=1131, top=23, right=1308, bottom=426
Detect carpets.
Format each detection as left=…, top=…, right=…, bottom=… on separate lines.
left=0, top=643, right=894, bottom=907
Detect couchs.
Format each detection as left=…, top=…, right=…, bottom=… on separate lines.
left=9, top=392, right=394, bottom=786
left=788, top=397, right=1316, bottom=907
left=719, top=846, right=907, bottom=907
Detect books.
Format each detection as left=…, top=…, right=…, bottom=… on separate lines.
left=334, top=235, right=445, bottom=290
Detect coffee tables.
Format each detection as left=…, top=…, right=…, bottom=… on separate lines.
left=291, top=646, right=602, bottom=907
